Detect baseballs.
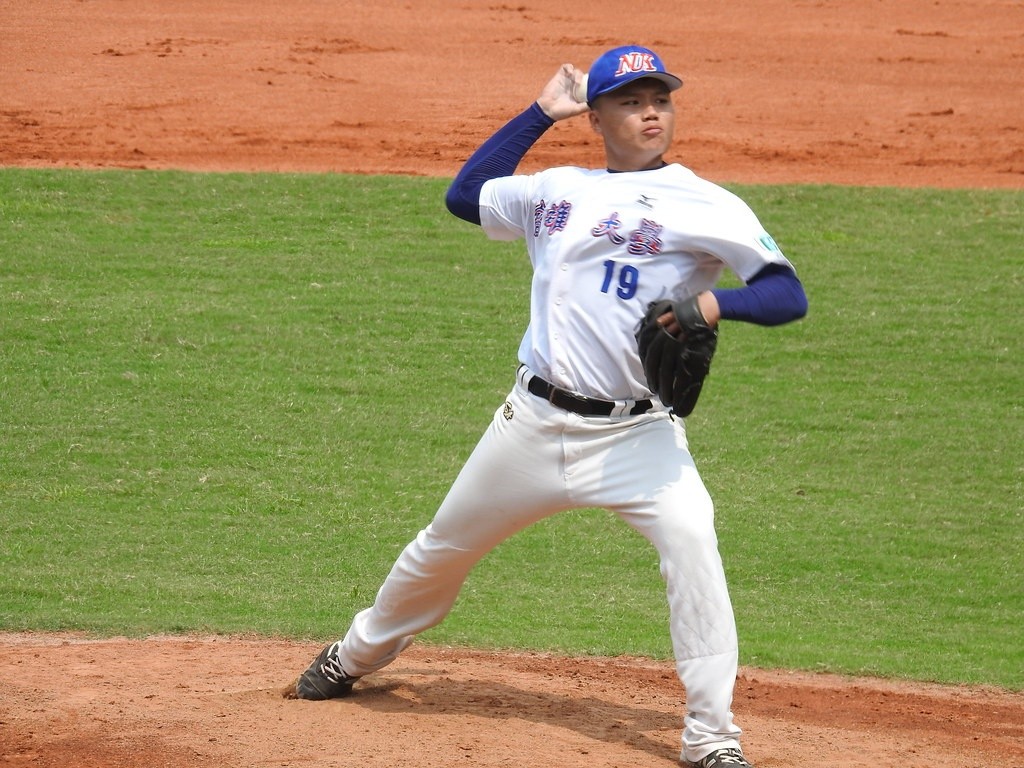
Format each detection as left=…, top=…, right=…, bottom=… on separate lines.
left=572, top=73, right=589, bottom=104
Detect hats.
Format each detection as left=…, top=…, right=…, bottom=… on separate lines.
left=585, top=45, right=683, bottom=106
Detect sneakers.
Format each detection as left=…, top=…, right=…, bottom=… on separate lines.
left=685, top=748, right=752, bottom=768
left=296, top=639, right=362, bottom=700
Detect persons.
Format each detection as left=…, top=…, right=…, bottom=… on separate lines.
left=293, top=45, right=808, bottom=768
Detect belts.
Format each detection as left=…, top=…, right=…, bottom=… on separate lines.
left=517, top=363, right=652, bottom=416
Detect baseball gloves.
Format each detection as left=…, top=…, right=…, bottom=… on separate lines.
left=633, top=290, right=719, bottom=418
